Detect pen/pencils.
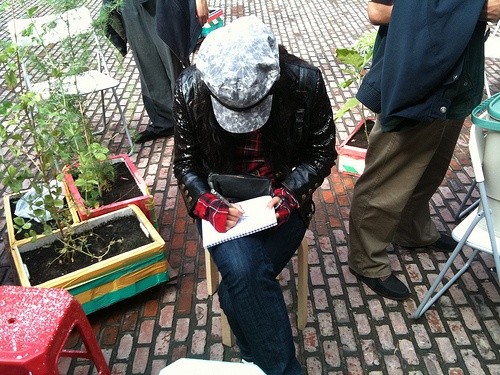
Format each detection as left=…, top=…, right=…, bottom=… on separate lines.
left=211, top=189, right=246, bottom=220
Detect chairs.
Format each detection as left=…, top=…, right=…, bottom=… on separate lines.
left=483, top=19, right=500, bottom=98
left=412, top=110, right=500, bottom=320
left=8, top=6, right=135, bottom=173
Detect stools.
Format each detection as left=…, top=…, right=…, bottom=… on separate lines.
left=0, top=285, right=109, bottom=375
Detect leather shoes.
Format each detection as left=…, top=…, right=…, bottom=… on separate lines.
left=349, top=267, right=409, bottom=300
left=427, top=234, right=457, bottom=251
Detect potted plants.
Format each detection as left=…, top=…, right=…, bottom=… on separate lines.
left=0, top=0, right=169, bottom=316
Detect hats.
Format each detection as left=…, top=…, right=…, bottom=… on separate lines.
left=196, top=14, right=279, bottom=134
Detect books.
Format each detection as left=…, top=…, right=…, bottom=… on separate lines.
left=202, top=195, right=278, bottom=250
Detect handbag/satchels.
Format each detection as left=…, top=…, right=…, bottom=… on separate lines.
left=208, top=173, right=273, bottom=201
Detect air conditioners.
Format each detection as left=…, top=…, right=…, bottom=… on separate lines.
left=333, top=28, right=377, bottom=176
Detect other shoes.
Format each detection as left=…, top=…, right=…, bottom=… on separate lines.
left=132, top=130, right=170, bottom=144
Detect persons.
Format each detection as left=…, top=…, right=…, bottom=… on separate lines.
left=175, top=14, right=338, bottom=374
left=99, top=0, right=209, bottom=143
left=349, top=0, right=500, bottom=300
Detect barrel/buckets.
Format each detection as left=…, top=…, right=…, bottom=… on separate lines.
left=470, top=91, right=500, bottom=201
